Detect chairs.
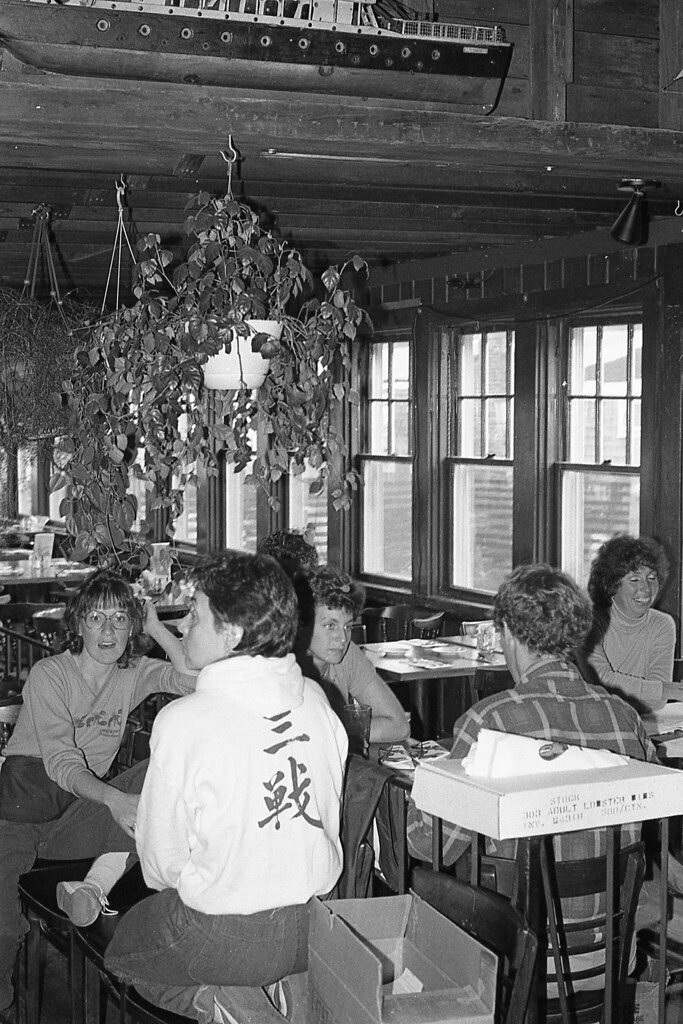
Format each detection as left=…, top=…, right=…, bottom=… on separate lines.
left=0, top=585, right=683, bottom=1024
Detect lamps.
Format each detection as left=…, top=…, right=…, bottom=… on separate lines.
left=610, top=178, right=660, bottom=246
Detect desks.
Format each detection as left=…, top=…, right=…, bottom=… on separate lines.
left=52, top=588, right=188, bottom=613
left=0, top=548, right=97, bottom=583
left=358, top=634, right=508, bottom=740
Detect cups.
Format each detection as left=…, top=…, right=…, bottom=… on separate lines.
left=342, top=704, right=373, bottom=762
left=477, top=624, right=496, bottom=653
left=29, top=553, right=43, bottom=573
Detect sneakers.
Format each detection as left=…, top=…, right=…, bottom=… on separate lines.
left=57, top=879, right=118, bottom=927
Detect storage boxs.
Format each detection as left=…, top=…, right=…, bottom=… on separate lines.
left=307, top=887, right=500, bottom=1024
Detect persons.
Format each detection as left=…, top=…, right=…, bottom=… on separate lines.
left=0, top=569, right=200, bottom=1024
left=103, top=549, right=348, bottom=1024
left=256, top=529, right=683, bottom=1001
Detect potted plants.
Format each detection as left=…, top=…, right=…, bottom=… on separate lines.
left=0, top=153, right=375, bottom=578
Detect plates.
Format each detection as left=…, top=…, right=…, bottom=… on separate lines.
left=377, top=644, right=411, bottom=656
left=432, top=646, right=468, bottom=656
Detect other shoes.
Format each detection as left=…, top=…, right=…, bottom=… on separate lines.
left=265, top=971, right=307, bottom=1024
left=212, top=986, right=290, bottom=1024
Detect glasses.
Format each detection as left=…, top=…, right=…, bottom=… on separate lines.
left=79, top=610, right=134, bottom=630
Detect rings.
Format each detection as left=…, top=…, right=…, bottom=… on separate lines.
left=131, top=821, right=136, bottom=828
